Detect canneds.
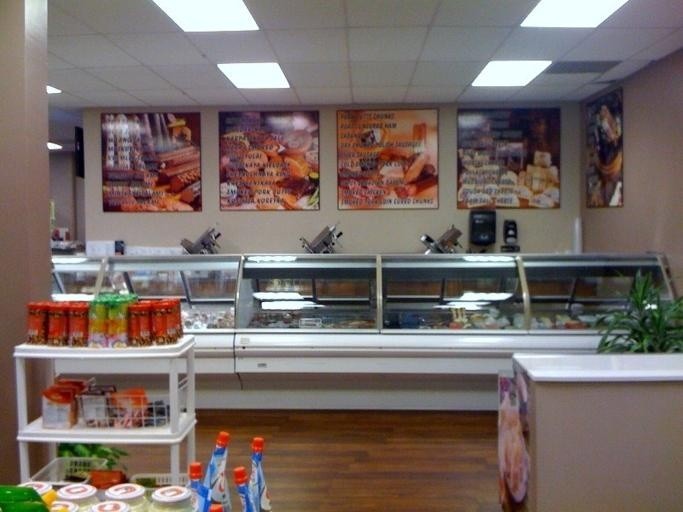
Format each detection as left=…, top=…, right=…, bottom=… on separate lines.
left=25, top=293, right=182, bottom=347
left=16, top=481, right=200, bottom=512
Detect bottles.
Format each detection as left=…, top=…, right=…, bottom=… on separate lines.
left=18, top=479, right=196, bottom=512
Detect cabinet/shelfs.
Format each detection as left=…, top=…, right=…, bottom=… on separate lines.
left=498, top=355, right=683, bottom=512
left=16, top=345, right=201, bottom=512
left=51, top=260, right=679, bottom=419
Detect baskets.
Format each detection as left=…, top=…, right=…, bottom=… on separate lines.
left=30, top=455, right=107, bottom=488
left=128, top=472, right=197, bottom=492
left=72, top=373, right=188, bottom=428
